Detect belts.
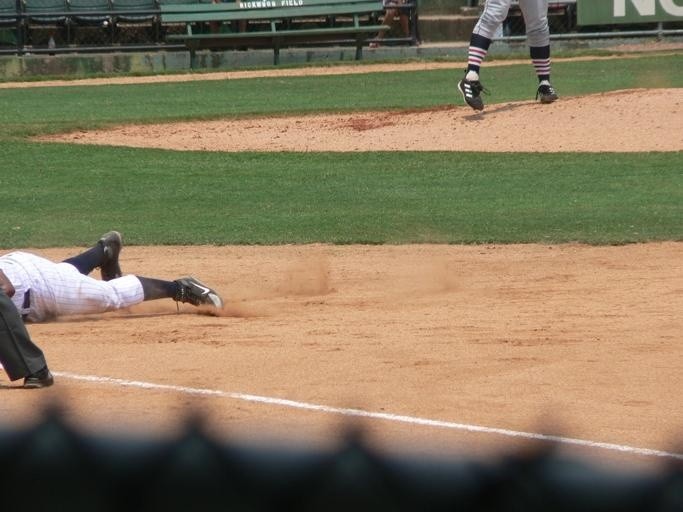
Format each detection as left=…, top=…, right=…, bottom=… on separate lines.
left=20, top=289, right=29, bottom=323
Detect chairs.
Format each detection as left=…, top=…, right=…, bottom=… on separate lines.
left=0, top=0, right=161, bottom=55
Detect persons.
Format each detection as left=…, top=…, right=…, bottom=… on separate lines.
left=1, top=231, right=225, bottom=322
left=369, top=0, right=410, bottom=49
left=1, top=291, right=53, bottom=388
left=457, top=0, right=559, bottom=112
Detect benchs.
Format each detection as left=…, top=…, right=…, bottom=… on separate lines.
left=157, top=0, right=391, bottom=68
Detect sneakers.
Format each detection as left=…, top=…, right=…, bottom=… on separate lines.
left=172, top=275, right=224, bottom=314
left=457, top=78, right=490, bottom=114
left=22, top=366, right=56, bottom=390
left=534, top=82, right=558, bottom=104
left=97, top=230, right=124, bottom=281
